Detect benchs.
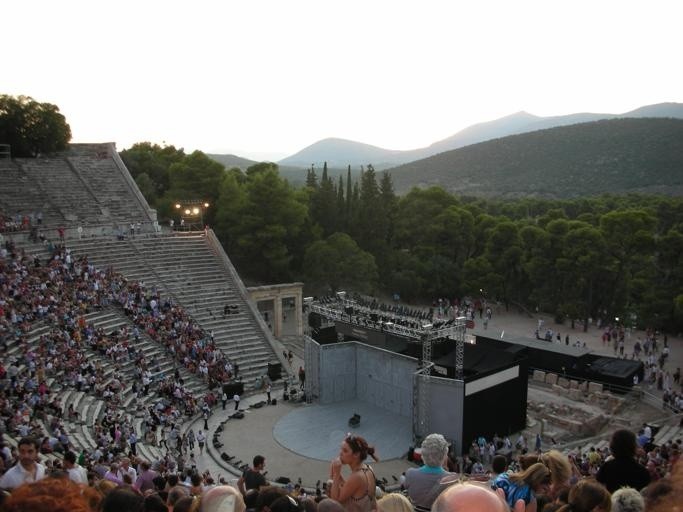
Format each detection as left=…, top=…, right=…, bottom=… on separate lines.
left=0, top=143, right=295, bottom=466
left=561, top=416, right=683, bottom=450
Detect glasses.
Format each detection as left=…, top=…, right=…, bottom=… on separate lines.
left=439, top=474, right=501, bottom=496
left=346, top=432, right=356, bottom=441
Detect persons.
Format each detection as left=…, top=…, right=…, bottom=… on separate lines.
left=535, top=315, right=683, bottom=413
left=0, top=210, right=65, bottom=243
left=436, top=295, right=493, bottom=328
left=0, top=240, right=245, bottom=510
left=78, top=224, right=83, bottom=240
left=237, top=424, right=683, bottom=512
left=118, top=217, right=185, bottom=240
left=256, top=350, right=305, bottom=401
